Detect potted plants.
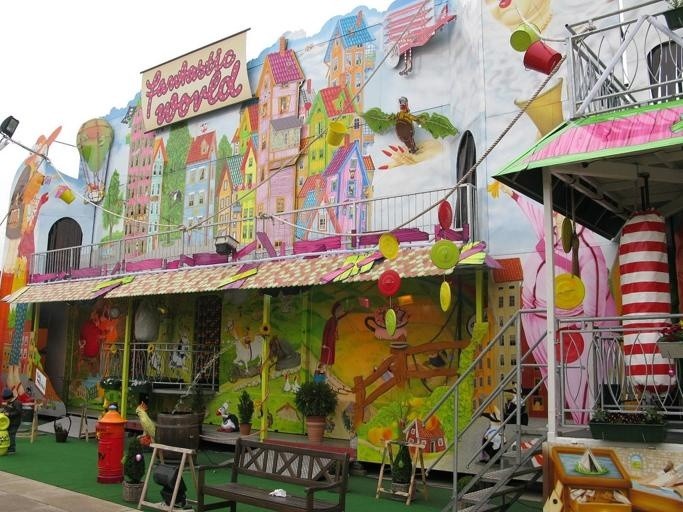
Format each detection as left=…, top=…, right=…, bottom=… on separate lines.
left=587, top=404, right=666, bottom=442
left=390, top=445, right=417, bottom=501
left=294, top=382, right=336, bottom=441
left=190, top=385, right=209, bottom=424
left=236, top=391, right=253, bottom=435
left=121, top=437, right=146, bottom=500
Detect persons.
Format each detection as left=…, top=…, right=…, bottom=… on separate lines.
left=0, top=387, right=23, bottom=455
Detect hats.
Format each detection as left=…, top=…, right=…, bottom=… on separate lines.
left=3, top=389, right=14, bottom=400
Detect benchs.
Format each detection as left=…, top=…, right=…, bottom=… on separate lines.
left=193, top=438, right=348, bottom=510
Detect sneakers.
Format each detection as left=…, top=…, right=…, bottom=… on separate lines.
left=7, top=447, right=16, bottom=454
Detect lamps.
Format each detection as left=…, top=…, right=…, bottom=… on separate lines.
left=0, top=117, right=48, bottom=162
left=214, top=234, right=239, bottom=257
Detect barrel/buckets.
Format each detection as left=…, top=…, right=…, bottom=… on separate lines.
left=522, top=38, right=561, bottom=73
left=510, top=22, right=543, bottom=52
left=327, top=121, right=349, bottom=144
left=155, top=411, right=202, bottom=469
left=513, top=77, right=563, bottom=136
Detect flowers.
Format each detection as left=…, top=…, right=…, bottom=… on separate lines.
left=657, top=318, right=683, bottom=341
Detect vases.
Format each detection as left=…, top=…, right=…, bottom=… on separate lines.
left=656, top=342, right=683, bottom=358
